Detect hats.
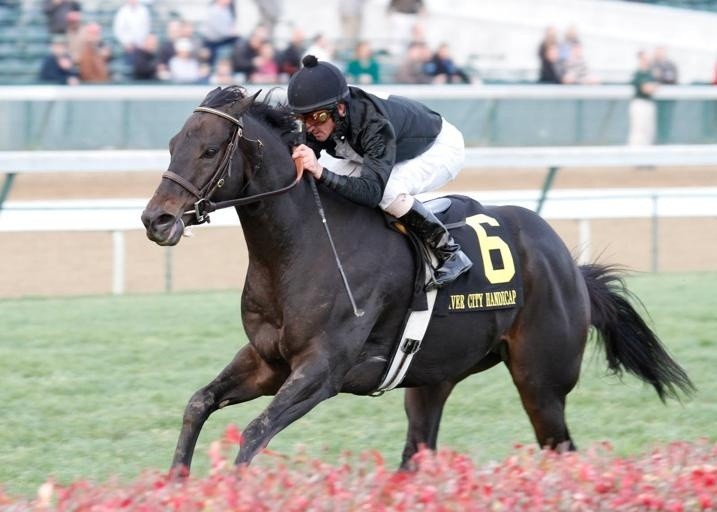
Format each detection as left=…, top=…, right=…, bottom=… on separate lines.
left=283, top=54, right=351, bottom=115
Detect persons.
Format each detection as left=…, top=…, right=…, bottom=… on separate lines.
left=627, top=50, right=656, bottom=170
left=285, top=55, right=474, bottom=292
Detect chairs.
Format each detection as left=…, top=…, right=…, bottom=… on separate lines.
left=2, top=4, right=714, bottom=84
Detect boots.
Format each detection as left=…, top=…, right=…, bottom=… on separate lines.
left=395, top=196, right=474, bottom=293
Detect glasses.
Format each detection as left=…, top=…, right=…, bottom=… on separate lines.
left=291, top=108, right=335, bottom=127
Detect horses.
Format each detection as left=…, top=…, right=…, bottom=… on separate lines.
left=141, top=84, right=699, bottom=481
